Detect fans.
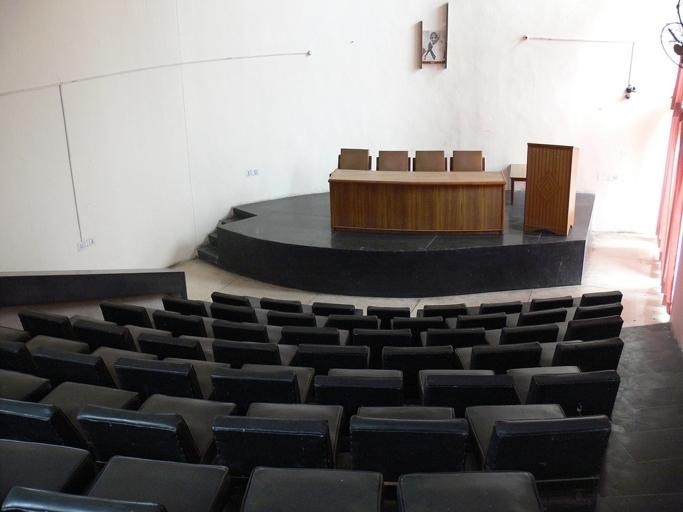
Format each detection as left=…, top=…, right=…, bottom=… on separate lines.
left=660, top=22, right=683, bottom=69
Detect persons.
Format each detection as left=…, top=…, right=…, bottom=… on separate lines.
left=424, top=32, right=441, bottom=60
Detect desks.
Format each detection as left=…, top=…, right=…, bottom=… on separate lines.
left=327, top=168, right=506, bottom=237
left=508, top=163, right=527, bottom=206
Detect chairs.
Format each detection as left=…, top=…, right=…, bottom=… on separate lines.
left=413, top=151, right=447, bottom=172
left=375, top=150, right=410, bottom=171
left=0, top=292, right=623, bottom=510
left=449, top=150, right=485, bottom=171
left=337, top=148, right=372, bottom=171
